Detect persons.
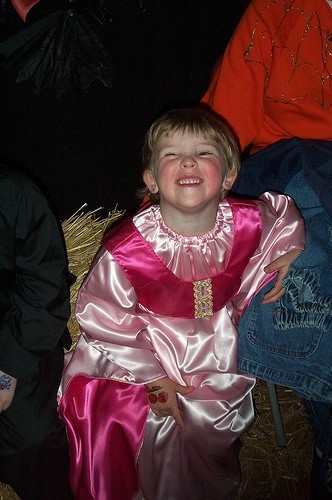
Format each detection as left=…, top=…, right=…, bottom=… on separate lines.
left=0, top=157, right=75, bottom=499
left=138, top=1, right=330, bottom=500
left=56, top=107, right=306, bottom=500
